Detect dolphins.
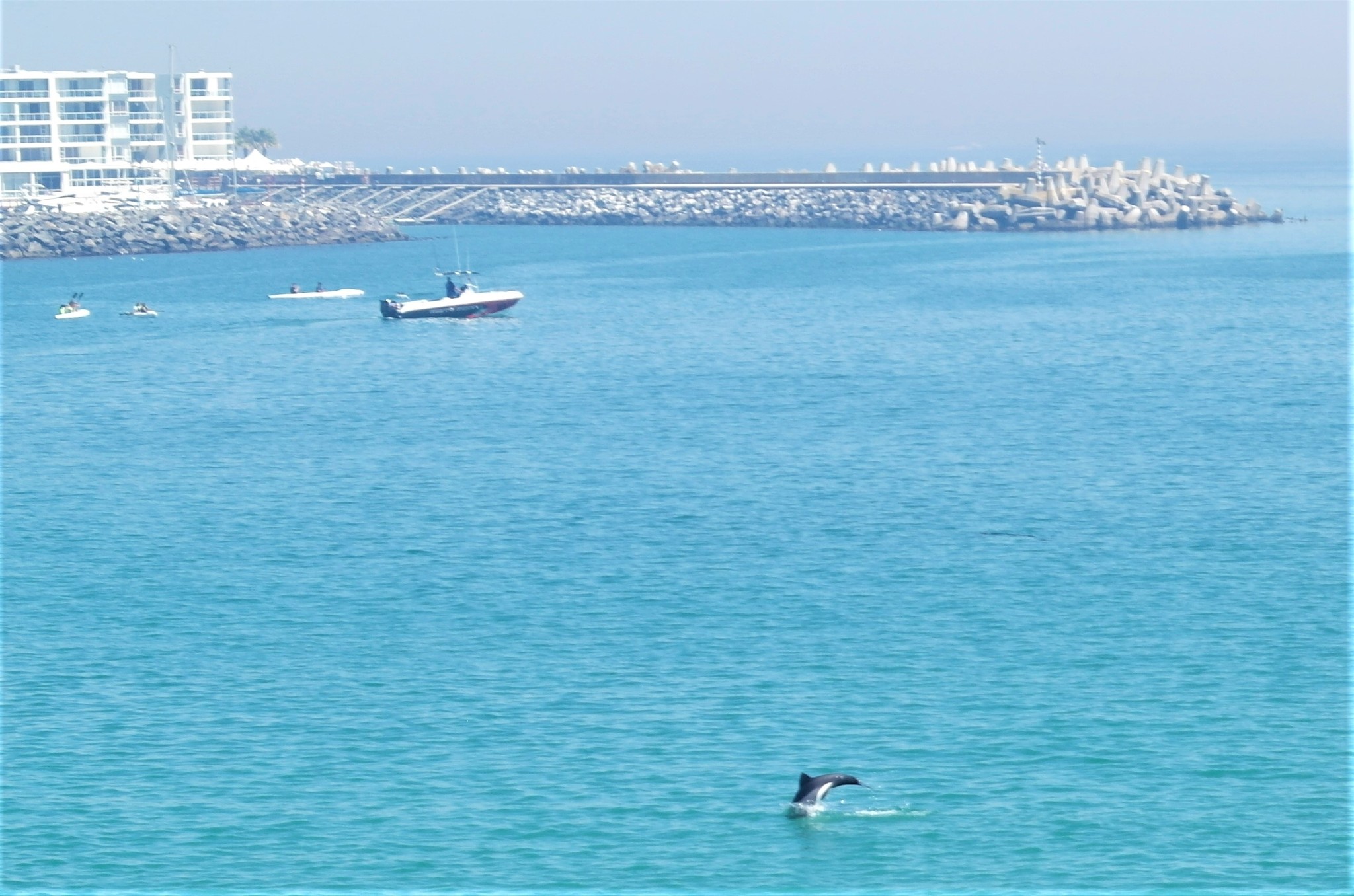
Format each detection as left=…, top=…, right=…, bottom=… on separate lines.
left=789, top=771, right=860, bottom=807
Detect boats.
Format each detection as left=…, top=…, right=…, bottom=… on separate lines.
left=378, top=270, right=525, bottom=319
left=132, top=305, right=158, bottom=318
left=55, top=308, right=91, bottom=320
left=267, top=288, right=366, bottom=300
